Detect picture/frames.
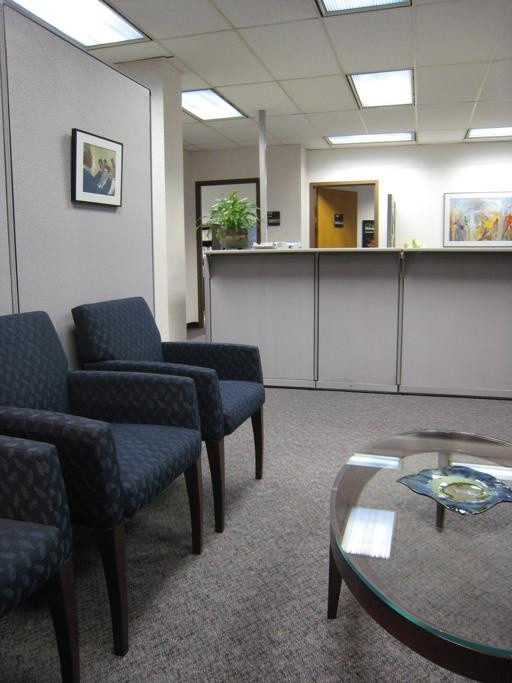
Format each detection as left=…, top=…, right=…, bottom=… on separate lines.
left=443, top=192, right=512, bottom=247
left=70, top=128, right=124, bottom=207
left=362, top=220, right=376, bottom=248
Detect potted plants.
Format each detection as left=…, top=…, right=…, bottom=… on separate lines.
left=443, top=192, right=512, bottom=247
left=362, top=220, right=376, bottom=248
left=194, top=190, right=263, bottom=249
left=70, top=128, right=124, bottom=207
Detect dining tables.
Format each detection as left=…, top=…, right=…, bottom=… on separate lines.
left=194, top=190, right=263, bottom=249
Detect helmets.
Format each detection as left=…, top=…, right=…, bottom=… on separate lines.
left=395, top=465, right=511, bottom=516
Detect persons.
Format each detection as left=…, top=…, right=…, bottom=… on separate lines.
left=83, top=143, right=115, bottom=195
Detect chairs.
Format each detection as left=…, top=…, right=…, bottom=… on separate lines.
left=70, top=298, right=266, bottom=535
left=4, top=297, right=203, bottom=655
left=3, top=435, right=81, bottom=683
